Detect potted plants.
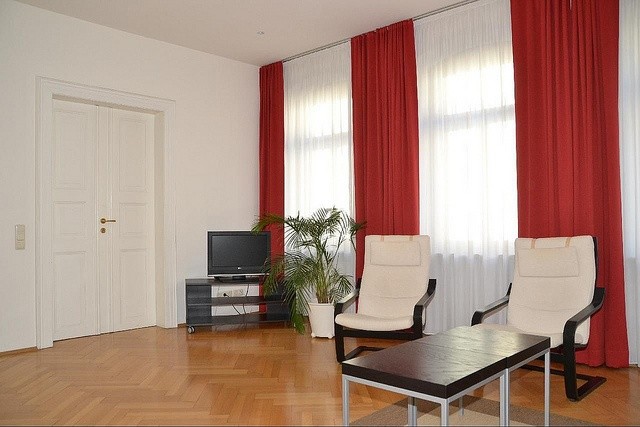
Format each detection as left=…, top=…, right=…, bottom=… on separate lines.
left=250, top=207, right=366, bottom=339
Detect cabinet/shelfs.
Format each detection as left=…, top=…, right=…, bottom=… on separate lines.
left=185, top=278, right=294, bottom=334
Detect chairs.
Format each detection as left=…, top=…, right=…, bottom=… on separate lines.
left=471, top=235, right=607, bottom=402
left=334, top=235, right=437, bottom=361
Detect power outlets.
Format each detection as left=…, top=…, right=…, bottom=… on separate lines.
left=217, top=291, right=232, bottom=298
left=238, top=289, right=247, bottom=297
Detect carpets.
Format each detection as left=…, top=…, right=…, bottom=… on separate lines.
left=349, top=394, right=608, bottom=426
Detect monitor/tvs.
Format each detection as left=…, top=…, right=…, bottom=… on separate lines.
left=208, top=230, right=271, bottom=283
